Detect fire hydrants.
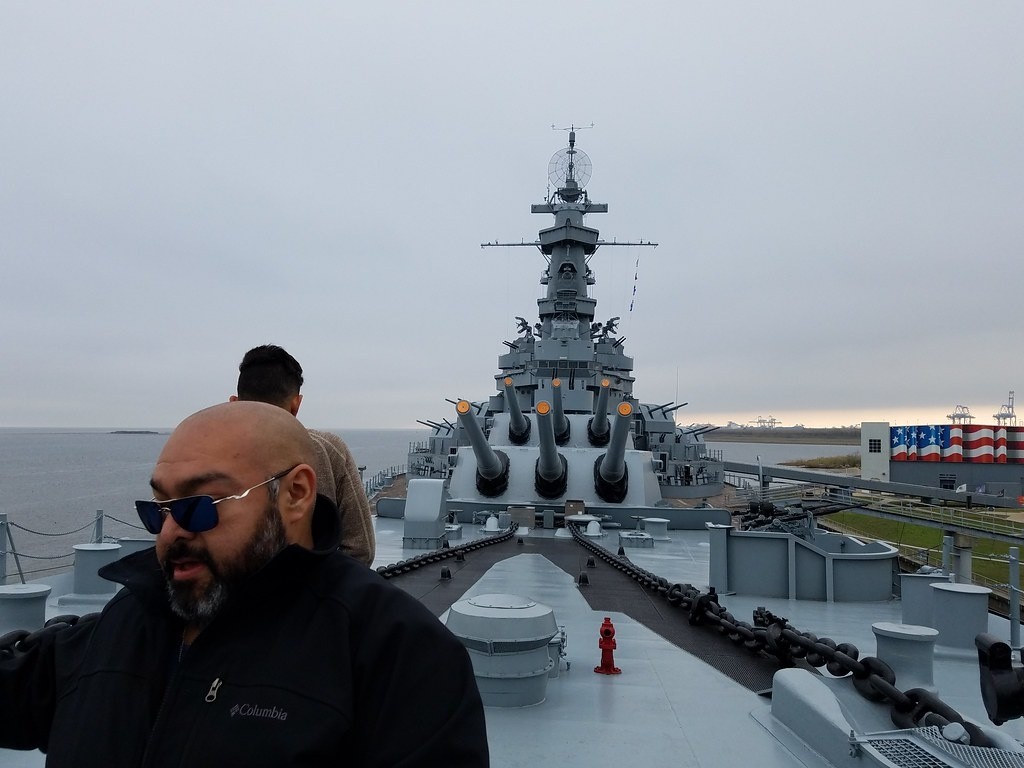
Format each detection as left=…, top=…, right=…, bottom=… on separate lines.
left=593, top=617, right=623, bottom=675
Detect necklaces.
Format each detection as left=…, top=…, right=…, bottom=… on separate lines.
left=179, top=628, right=187, bottom=662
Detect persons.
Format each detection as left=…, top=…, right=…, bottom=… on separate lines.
left=230, top=345, right=376, bottom=568
left=0, top=401, right=490, bottom=768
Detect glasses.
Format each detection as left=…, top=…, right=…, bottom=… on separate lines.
left=134, top=464, right=300, bottom=534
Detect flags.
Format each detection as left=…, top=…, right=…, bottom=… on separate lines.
left=956, top=483, right=966, bottom=493
left=1018, top=496, right=1024, bottom=504
left=997, top=489, right=1005, bottom=497
left=975, top=484, right=985, bottom=495
